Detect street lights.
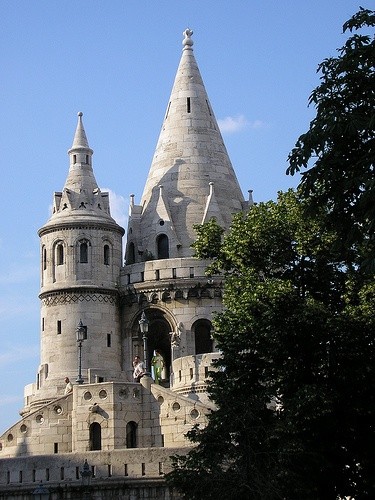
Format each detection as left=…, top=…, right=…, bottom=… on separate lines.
left=74, top=318, right=86, bottom=385
left=138, top=309, right=152, bottom=378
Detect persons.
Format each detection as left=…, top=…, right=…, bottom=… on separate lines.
left=131, top=348, right=166, bottom=384
left=63, top=376, right=74, bottom=397
